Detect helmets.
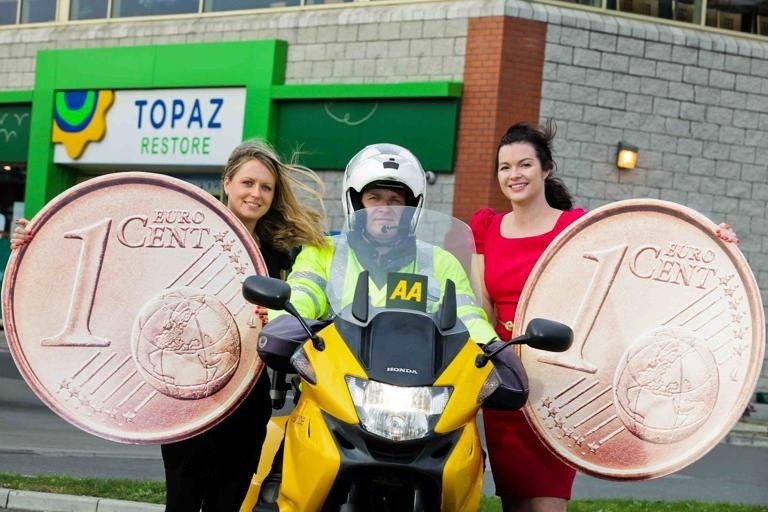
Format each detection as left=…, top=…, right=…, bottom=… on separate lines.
left=341, top=143, right=427, bottom=237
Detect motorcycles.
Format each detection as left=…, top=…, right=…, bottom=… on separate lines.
left=240, top=201, right=577, bottom=510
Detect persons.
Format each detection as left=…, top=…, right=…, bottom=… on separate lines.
left=462, top=115, right=740, bottom=511
left=8, top=137, right=333, bottom=511
left=250, top=140, right=507, bottom=510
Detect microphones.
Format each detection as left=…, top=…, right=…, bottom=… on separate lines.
left=382, top=225, right=407, bottom=231
